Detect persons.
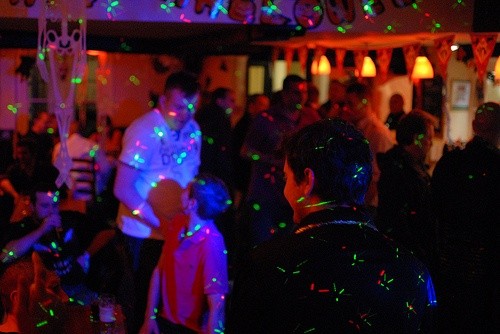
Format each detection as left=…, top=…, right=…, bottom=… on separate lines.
left=136, top=178, right=233, bottom=334
left=374, top=107, right=437, bottom=236
left=6, top=178, right=117, bottom=309
left=16, top=106, right=121, bottom=212
left=425, top=103, right=500, bottom=231
left=190, top=73, right=427, bottom=208
left=228, top=117, right=438, bottom=334
left=111, top=70, right=204, bottom=330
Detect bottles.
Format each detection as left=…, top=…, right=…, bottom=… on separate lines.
left=98, top=294, right=115, bottom=323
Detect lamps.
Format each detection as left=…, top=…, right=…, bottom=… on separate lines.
left=412, top=47, right=434, bottom=79
